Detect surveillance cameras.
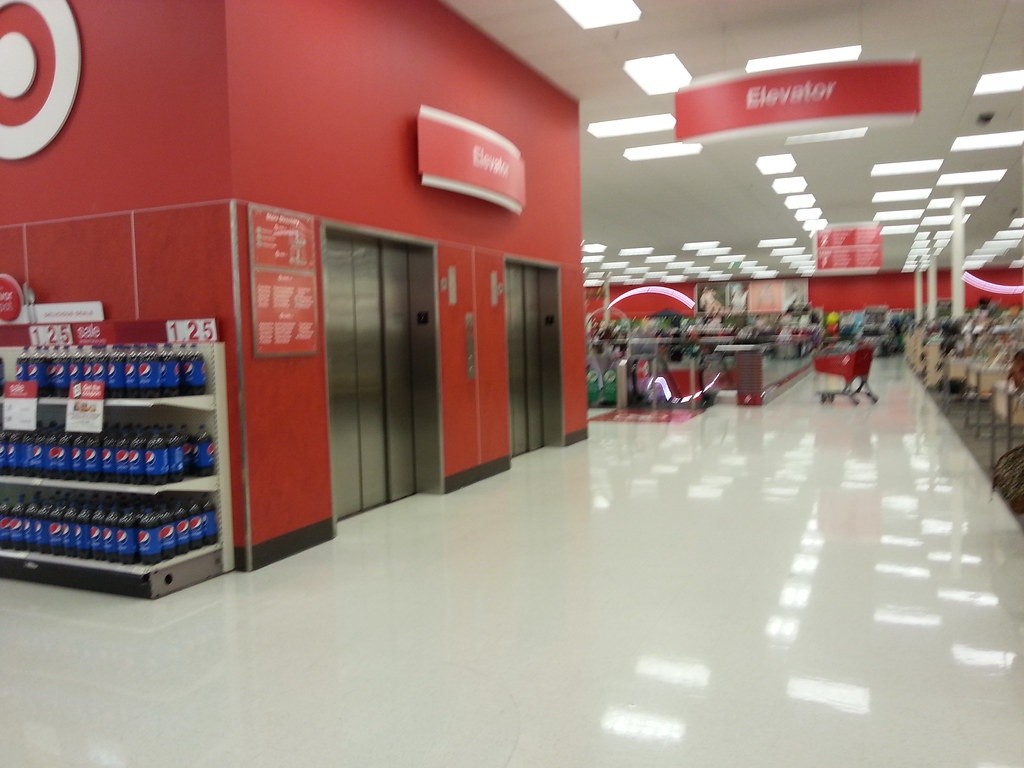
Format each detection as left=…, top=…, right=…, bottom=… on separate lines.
left=979, top=113, right=994, bottom=122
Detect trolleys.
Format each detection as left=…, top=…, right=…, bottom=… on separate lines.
left=814, top=342, right=882, bottom=406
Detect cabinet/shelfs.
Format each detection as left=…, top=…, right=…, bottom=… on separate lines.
left=596, top=305, right=1024, bottom=473
left=0, top=344, right=236, bottom=600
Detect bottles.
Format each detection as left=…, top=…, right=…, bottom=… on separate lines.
left=1, top=490, right=90, bottom=560
left=95, top=347, right=208, bottom=399
left=103, top=422, right=217, bottom=483
left=90, top=493, right=219, bottom=567
left=15, top=341, right=95, bottom=399
left=0, top=420, right=101, bottom=482
